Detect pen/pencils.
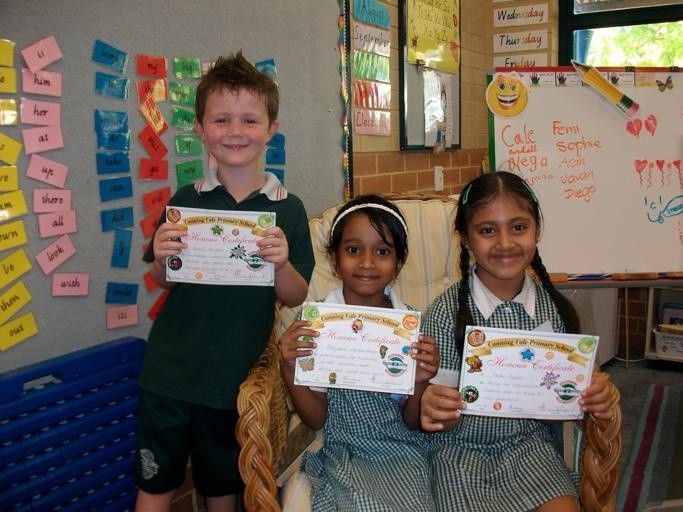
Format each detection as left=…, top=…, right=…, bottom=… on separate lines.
left=572, top=61, right=640, bottom=119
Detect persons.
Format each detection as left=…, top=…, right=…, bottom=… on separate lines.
left=417, top=169, right=615, bottom=511
left=132, top=46, right=315, bottom=511
left=278, top=192, right=440, bottom=511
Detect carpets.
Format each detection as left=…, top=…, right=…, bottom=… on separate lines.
left=616, top=383, right=683, bottom=511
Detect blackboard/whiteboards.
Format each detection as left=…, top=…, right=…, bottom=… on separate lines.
left=488, top=67, right=683, bottom=289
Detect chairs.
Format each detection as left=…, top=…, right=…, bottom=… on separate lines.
left=233, top=187, right=622, bottom=511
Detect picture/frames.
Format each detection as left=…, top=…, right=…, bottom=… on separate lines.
left=396, top=0, right=462, bottom=153
